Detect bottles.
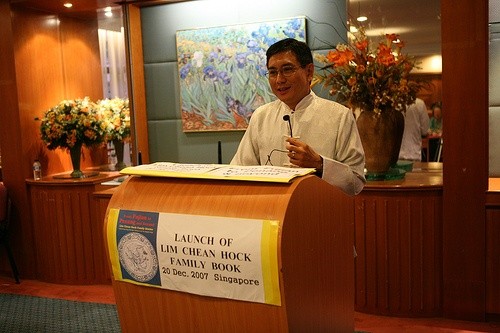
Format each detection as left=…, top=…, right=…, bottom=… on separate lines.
left=33, top=160, right=42, bottom=180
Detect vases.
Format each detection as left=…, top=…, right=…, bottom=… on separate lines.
left=356, top=107, right=405, bottom=176
left=113, top=137, right=126, bottom=171
left=70, top=143, right=87, bottom=180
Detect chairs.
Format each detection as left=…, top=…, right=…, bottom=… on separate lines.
left=0, top=191, right=21, bottom=284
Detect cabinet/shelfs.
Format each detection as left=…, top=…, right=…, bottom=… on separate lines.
left=25, top=170, right=122, bottom=285
left=354, top=174, right=442, bottom=319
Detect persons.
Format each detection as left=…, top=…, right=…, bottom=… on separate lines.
left=396, top=97, right=434, bottom=162
left=229, top=38, right=366, bottom=197
left=430, top=102, right=444, bottom=163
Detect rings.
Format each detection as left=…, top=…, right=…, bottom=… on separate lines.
left=293, top=153, right=295, bottom=158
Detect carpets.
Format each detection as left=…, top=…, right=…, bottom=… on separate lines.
left=0, top=293, right=122, bottom=333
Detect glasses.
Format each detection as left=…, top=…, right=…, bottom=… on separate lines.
left=264, top=65, right=306, bottom=79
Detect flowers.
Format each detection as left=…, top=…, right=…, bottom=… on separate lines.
left=97, top=97, right=132, bottom=146
left=34, top=96, right=109, bottom=153
left=306, top=0, right=422, bottom=122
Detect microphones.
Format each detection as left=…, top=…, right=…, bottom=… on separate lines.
left=265, top=114, right=293, bottom=165
